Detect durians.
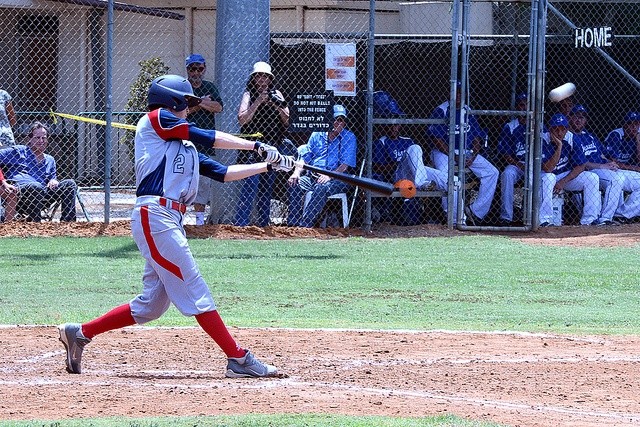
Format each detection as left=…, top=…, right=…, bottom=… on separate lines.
left=268, top=89, right=288, bottom=109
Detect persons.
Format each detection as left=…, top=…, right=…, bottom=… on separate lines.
left=429, top=80, right=499, bottom=229
left=58, top=76, right=295, bottom=379
left=235, top=62, right=289, bottom=228
left=185, top=54, right=223, bottom=226
left=568, top=105, right=640, bottom=225
left=538, top=114, right=602, bottom=226
left=373, top=113, right=467, bottom=228
left=0, top=89, right=16, bottom=223
left=497, top=93, right=549, bottom=226
left=0, top=121, right=78, bottom=222
left=603, top=112, right=640, bottom=224
left=287, top=105, right=357, bottom=227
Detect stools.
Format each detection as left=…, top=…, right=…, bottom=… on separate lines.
left=159, top=198, right=186, bottom=214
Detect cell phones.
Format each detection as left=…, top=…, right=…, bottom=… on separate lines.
left=265, top=85, right=287, bottom=109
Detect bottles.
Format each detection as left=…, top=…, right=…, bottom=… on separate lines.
left=250, top=62, right=275, bottom=79
left=186, top=54, right=206, bottom=68
left=515, top=93, right=530, bottom=103
left=624, top=111, right=640, bottom=122
left=571, top=105, right=587, bottom=114
left=550, top=113, right=568, bottom=127
left=384, top=101, right=404, bottom=117
left=334, top=104, right=347, bottom=119
left=456, top=79, right=470, bottom=88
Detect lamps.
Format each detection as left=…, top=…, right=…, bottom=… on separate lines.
left=299, top=188, right=352, bottom=229
left=0, top=174, right=92, bottom=222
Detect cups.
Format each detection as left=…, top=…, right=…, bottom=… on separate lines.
left=190, top=66, right=205, bottom=70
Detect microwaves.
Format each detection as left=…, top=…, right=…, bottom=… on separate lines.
left=262, top=150, right=394, bottom=196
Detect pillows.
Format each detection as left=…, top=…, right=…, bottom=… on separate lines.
left=146, top=74, right=202, bottom=111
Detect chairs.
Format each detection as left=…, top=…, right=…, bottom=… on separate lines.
left=226, top=349, right=277, bottom=377
left=466, top=204, right=481, bottom=227
left=57, top=323, right=91, bottom=374
left=612, top=215, right=632, bottom=225
left=606, top=221, right=618, bottom=225
left=591, top=219, right=606, bottom=226
left=541, top=221, right=554, bottom=227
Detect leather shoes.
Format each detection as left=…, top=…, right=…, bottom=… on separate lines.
left=267, top=151, right=296, bottom=174
left=255, top=142, right=282, bottom=164
